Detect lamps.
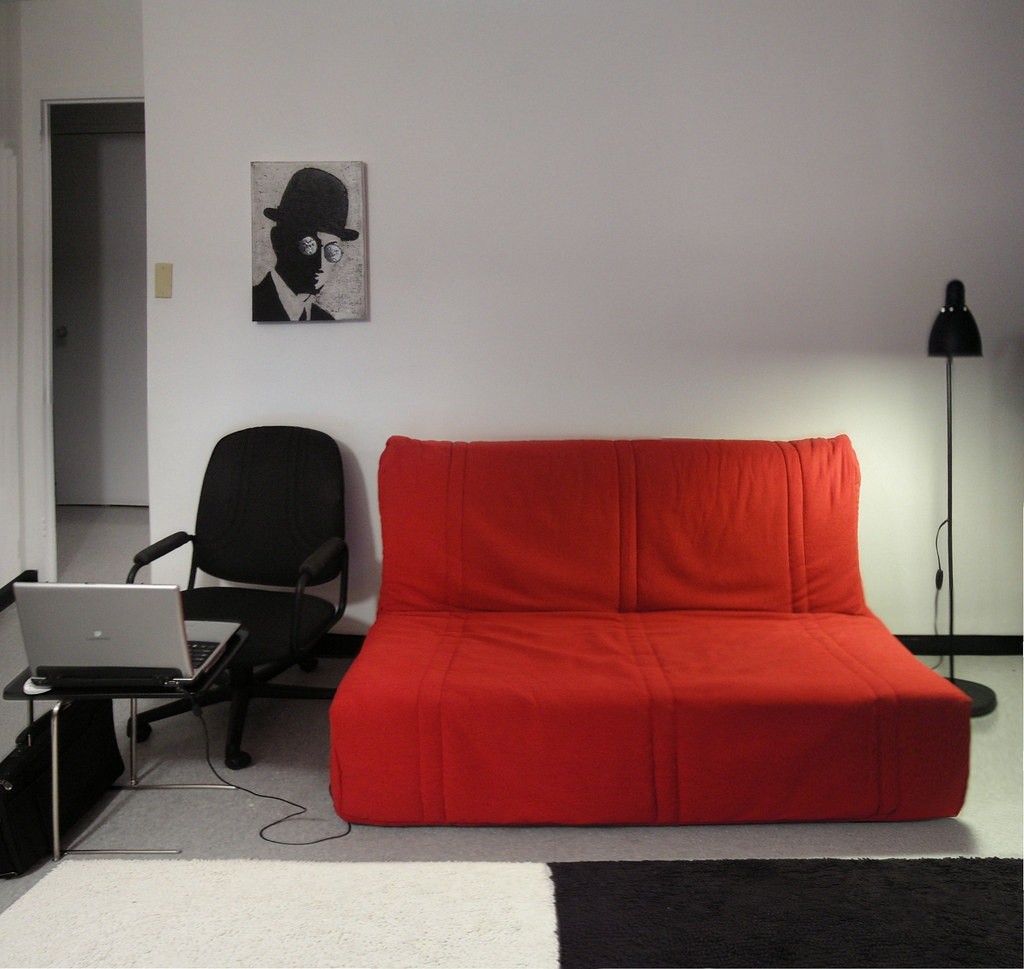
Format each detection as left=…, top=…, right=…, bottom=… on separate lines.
left=926, top=278, right=998, bottom=720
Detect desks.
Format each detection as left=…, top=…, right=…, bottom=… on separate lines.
left=2, top=631, right=249, bottom=863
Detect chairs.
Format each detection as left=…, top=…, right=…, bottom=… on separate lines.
left=125, top=425, right=349, bottom=772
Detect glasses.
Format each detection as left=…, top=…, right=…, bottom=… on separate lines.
left=282, top=236, right=343, bottom=263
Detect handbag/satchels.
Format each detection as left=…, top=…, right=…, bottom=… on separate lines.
left=0, top=697, right=126, bottom=879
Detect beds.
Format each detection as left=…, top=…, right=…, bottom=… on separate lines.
left=327, top=431, right=976, bottom=831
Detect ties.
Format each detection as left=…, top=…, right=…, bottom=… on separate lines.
left=299, top=308, right=307, bottom=322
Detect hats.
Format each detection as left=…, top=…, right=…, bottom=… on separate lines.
left=263, top=167, right=361, bottom=241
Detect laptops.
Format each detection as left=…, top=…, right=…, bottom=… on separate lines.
left=11, top=582, right=242, bottom=691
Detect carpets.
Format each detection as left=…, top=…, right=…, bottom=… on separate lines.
left=0, top=858, right=1024, bottom=969
